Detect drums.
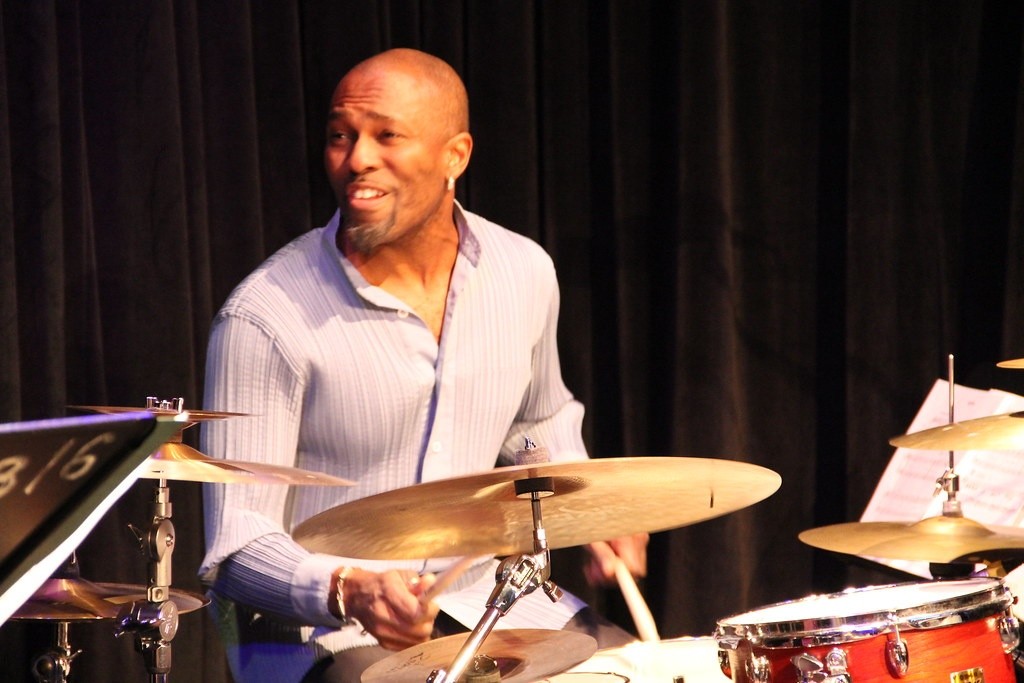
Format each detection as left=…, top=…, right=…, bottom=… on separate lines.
left=709, top=577, right=1020, bottom=683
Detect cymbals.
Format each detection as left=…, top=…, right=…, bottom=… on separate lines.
left=134, top=440, right=364, bottom=489
left=65, top=402, right=261, bottom=437
left=996, top=358, right=1024, bottom=371
left=10, top=576, right=215, bottom=626
left=359, top=626, right=599, bottom=683
left=797, top=513, right=1024, bottom=568
left=288, top=451, right=784, bottom=565
left=887, top=410, right=1024, bottom=454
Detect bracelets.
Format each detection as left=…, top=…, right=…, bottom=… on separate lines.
left=337, top=562, right=356, bottom=628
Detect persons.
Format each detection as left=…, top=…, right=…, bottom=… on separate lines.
left=196, top=50, right=651, bottom=683
